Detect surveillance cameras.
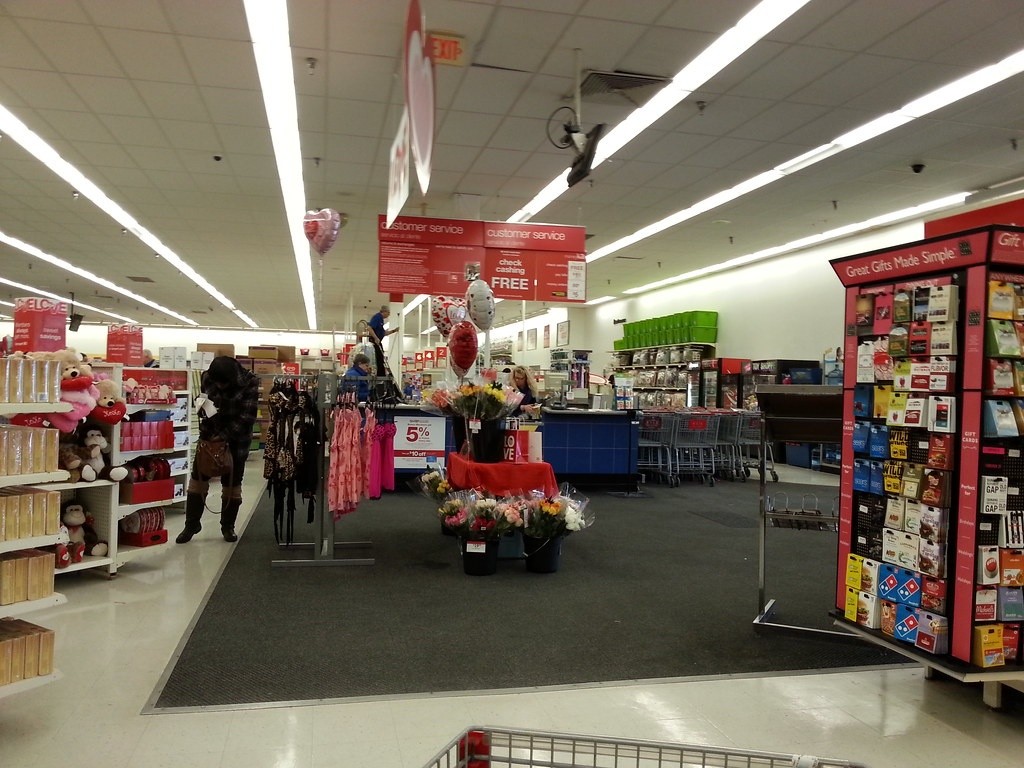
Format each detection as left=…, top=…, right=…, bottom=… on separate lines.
left=911, top=164, right=926, bottom=174
left=213, top=155, right=223, bottom=162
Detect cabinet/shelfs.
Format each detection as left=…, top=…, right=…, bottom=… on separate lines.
left=31, top=479, right=114, bottom=575
left=166, top=369, right=200, bottom=514
left=0, top=402, right=74, bottom=700
left=606, top=342, right=720, bottom=391
left=92, top=362, right=175, bottom=582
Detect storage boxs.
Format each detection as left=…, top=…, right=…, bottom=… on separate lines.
left=614, top=310, right=718, bottom=350
left=844, top=279, right=1024, bottom=668
left=0, top=343, right=296, bottom=687
left=789, top=367, right=822, bottom=385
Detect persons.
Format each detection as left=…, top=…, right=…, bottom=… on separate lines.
left=367, top=304, right=399, bottom=377
left=511, top=364, right=540, bottom=417
left=142, top=349, right=161, bottom=368
left=175, top=355, right=260, bottom=544
left=339, top=353, right=372, bottom=435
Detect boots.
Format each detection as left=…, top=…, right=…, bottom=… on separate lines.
left=219, top=500, right=241, bottom=542
left=175, top=492, right=206, bottom=544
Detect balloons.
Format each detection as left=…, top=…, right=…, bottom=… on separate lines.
left=431, top=279, right=497, bottom=378
left=303, top=206, right=341, bottom=258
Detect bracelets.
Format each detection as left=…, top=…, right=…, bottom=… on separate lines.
left=392, top=330, right=394, bottom=333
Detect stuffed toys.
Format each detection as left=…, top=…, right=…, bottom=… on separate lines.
left=2, top=348, right=129, bottom=570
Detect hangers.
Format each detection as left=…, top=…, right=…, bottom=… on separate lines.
left=272, top=373, right=397, bottom=425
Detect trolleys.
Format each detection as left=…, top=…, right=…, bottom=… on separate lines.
left=636, top=409, right=779, bottom=488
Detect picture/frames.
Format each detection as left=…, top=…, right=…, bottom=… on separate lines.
left=543, top=325, right=550, bottom=348
left=527, top=327, right=538, bottom=351
left=556, top=320, right=570, bottom=348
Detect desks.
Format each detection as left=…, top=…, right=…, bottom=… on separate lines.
left=446, top=452, right=559, bottom=560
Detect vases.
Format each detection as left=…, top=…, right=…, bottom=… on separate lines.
left=452, top=416, right=468, bottom=455
left=461, top=536, right=499, bottom=577
left=470, top=419, right=506, bottom=463
left=522, top=533, right=562, bottom=575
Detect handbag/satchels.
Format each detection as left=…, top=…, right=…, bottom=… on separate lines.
left=195, top=439, right=234, bottom=478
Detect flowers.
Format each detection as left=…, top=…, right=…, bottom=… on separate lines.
left=420, top=380, right=524, bottom=419
left=420, top=471, right=586, bottom=541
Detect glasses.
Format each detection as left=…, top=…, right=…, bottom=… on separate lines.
left=515, top=376, right=526, bottom=380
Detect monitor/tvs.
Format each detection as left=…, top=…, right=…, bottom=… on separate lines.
left=69, top=313, right=84, bottom=332
left=567, top=123, right=607, bottom=188
left=544, top=370, right=569, bottom=392
